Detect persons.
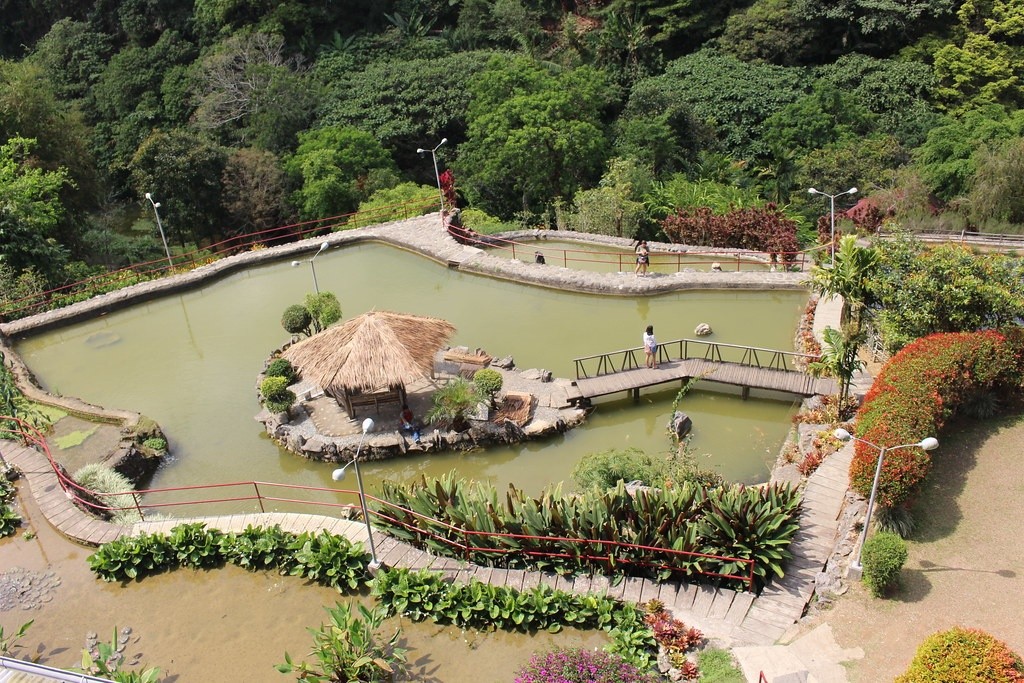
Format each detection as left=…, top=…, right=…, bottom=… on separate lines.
left=643, top=325, right=660, bottom=369
left=635, top=239, right=650, bottom=277
left=400, top=404, right=421, bottom=444
left=440, top=167, right=457, bottom=208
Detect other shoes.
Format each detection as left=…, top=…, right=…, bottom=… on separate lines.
left=653, top=366, right=660, bottom=369
left=647, top=366, right=652, bottom=369
left=420, top=432, right=423, bottom=435
left=416, top=438, right=420, bottom=443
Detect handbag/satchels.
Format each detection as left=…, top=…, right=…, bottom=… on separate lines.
left=638, top=255, right=646, bottom=263
left=403, top=422, right=414, bottom=431
left=651, top=347, right=656, bottom=352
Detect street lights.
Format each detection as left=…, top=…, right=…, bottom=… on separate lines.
left=332, top=416, right=389, bottom=578
left=417, top=138, right=448, bottom=217
left=832, top=427, right=939, bottom=585
left=146, top=193, right=177, bottom=276
left=291, top=242, right=329, bottom=318
left=807, top=186, right=858, bottom=271
